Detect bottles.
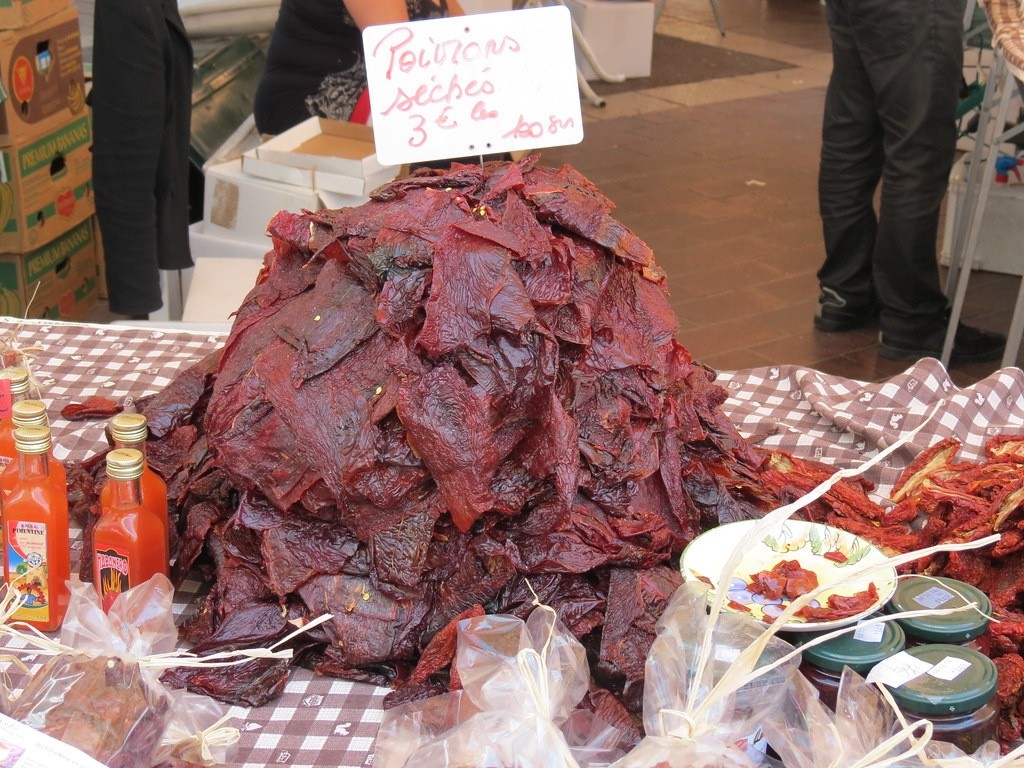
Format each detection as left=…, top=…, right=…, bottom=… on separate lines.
left=0, top=365, right=70, bottom=632
left=91, top=448, right=167, bottom=618
left=100, top=412, right=169, bottom=583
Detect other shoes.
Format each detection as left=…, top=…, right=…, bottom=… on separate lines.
left=876, top=321, right=1007, bottom=364
left=814, top=284, right=882, bottom=332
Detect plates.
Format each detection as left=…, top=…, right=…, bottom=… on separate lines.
left=680, top=518, right=899, bottom=632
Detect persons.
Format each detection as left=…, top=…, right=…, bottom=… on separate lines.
left=811, top=0, right=1010, bottom=366
left=253, top=0, right=467, bottom=144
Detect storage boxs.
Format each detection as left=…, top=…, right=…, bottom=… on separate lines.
left=149, top=112, right=411, bottom=323
left=0, top=0, right=112, bottom=324
left=938, top=151, right=1024, bottom=277
left=549, top=0, right=654, bottom=80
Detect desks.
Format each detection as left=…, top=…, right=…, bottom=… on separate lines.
left=942, top=0, right=1024, bottom=373
left=1, top=317, right=1024, bottom=768
